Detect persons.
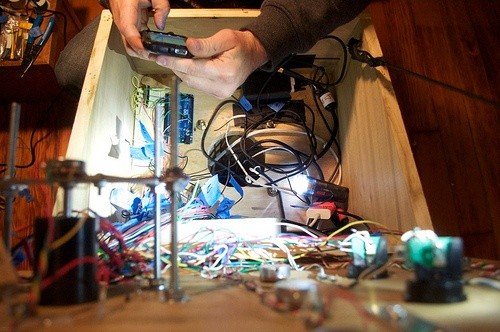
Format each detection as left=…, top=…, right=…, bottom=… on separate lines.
left=54, top=0, right=379, bottom=97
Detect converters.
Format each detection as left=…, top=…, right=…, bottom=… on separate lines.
left=244, top=71, right=307, bottom=101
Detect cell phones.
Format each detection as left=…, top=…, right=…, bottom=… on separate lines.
left=141, top=29, right=194, bottom=58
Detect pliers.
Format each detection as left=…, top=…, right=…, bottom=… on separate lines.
left=18, top=14, right=56, bottom=81
